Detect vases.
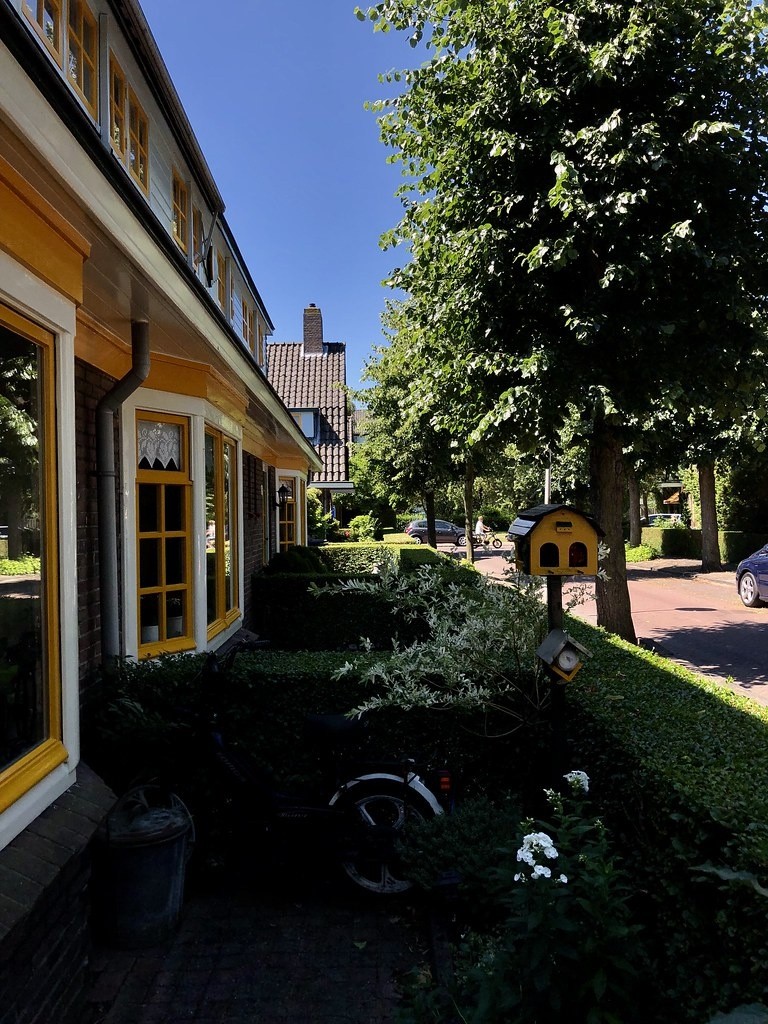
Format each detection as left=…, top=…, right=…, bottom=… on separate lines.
left=167, top=617, right=184, bottom=637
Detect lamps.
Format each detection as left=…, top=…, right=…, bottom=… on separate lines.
left=272, top=483, right=288, bottom=512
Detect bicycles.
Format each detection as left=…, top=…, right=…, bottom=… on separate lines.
left=471, top=529, right=502, bottom=549
left=124, top=635, right=453, bottom=904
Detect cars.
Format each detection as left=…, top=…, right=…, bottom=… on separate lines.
left=641, top=514, right=681, bottom=525
left=737, top=541, right=768, bottom=606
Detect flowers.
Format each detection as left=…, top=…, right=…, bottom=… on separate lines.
left=166, top=597, right=181, bottom=618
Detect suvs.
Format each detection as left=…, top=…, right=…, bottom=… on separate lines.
left=404, top=519, right=467, bottom=546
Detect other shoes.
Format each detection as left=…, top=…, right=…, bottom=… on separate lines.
left=481, top=542, right=486, bottom=546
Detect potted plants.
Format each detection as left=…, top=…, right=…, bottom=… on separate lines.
left=140, top=595, right=160, bottom=645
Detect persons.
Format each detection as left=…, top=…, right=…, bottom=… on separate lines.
left=205, top=520, right=216, bottom=549
left=475, top=516, right=490, bottom=546
